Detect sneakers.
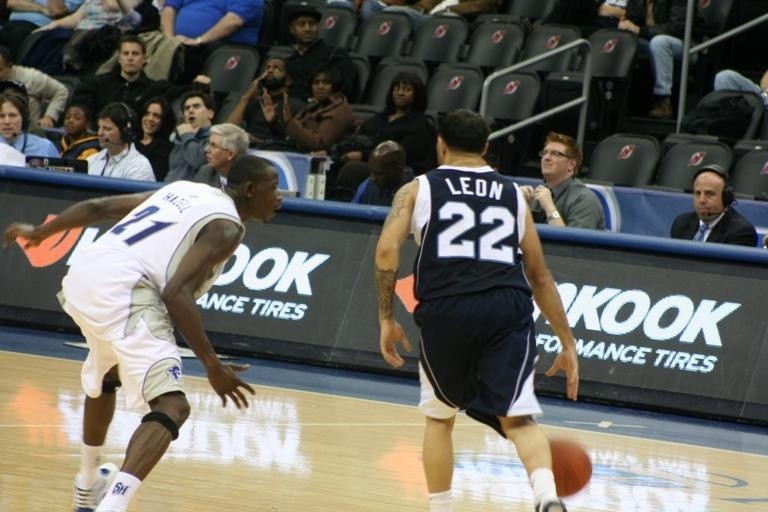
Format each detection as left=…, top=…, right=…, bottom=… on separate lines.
left=74, top=458, right=120, bottom=512
left=649, top=95, right=674, bottom=117
left=535, top=495, right=565, bottom=512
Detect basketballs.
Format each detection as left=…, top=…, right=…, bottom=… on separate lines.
left=550, top=439, right=591, bottom=495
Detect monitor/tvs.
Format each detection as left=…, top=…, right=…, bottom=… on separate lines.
left=25, top=156, right=88, bottom=175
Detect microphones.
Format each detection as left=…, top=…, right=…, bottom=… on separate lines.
left=12, top=132, right=26, bottom=137
left=708, top=202, right=738, bottom=216
left=105, top=138, right=125, bottom=145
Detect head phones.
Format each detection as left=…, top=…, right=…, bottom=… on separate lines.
left=120, top=101, right=141, bottom=143
left=691, top=164, right=735, bottom=208
left=22, top=114, right=31, bottom=131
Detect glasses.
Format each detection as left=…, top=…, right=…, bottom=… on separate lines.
left=204, top=141, right=230, bottom=152
left=537, top=148, right=573, bottom=161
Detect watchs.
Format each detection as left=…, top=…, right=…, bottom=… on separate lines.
left=547, top=210, right=560, bottom=222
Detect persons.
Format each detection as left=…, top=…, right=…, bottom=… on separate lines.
left=617, top=1, right=711, bottom=120
left=3, top=154, right=282, bottom=511
left=671, top=164, right=758, bottom=248
left=519, top=130, right=606, bottom=232
left=713, top=68, right=767, bottom=106
left=373, top=108, right=579, bottom=512
left=594, top=1, right=630, bottom=32
left=2, top=2, right=502, bottom=207
